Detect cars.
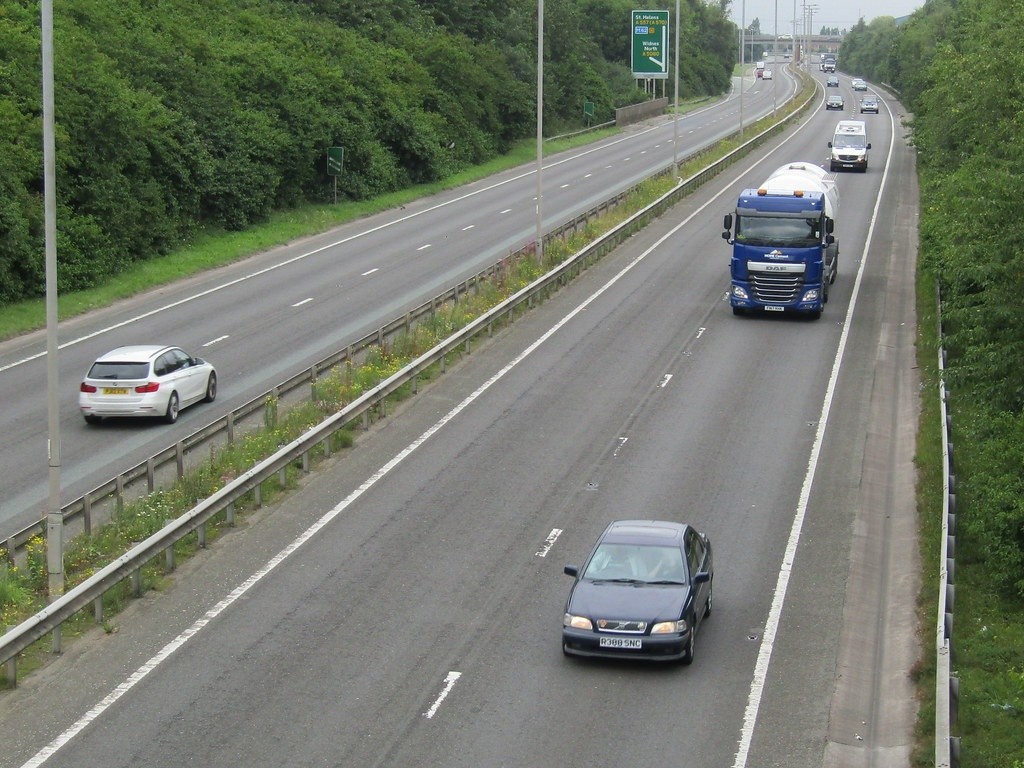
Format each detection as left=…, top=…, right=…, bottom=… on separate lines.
left=79, top=345, right=218, bottom=424
left=851, top=78, right=863, bottom=87
left=859, top=94, right=880, bottom=115
left=855, top=81, right=866, bottom=91
left=757, top=70, right=764, bottom=77
left=826, top=76, right=840, bottom=87
left=824, top=95, right=845, bottom=110
left=559, top=519, right=715, bottom=665
left=756, top=51, right=803, bottom=69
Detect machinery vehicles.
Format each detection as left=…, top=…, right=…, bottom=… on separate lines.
left=720, top=160, right=842, bottom=319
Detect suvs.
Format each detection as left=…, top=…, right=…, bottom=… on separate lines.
left=778, top=34, right=793, bottom=40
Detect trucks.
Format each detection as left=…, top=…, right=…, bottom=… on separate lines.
left=763, top=70, right=772, bottom=80
left=818, top=54, right=836, bottom=73
left=827, top=120, right=873, bottom=172
